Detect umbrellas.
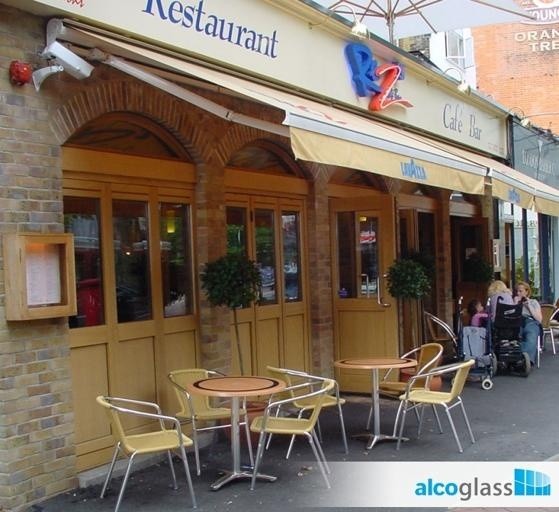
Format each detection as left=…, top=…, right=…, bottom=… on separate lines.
left=312, top=0, right=537, bottom=44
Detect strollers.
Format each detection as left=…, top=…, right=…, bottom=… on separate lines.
left=450, top=296, right=494, bottom=389
left=490, top=296, right=531, bottom=376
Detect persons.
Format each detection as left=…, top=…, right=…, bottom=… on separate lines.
left=482, top=280, right=516, bottom=322
left=513, top=281, right=542, bottom=377
left=467, top=298, right=488, bottom=327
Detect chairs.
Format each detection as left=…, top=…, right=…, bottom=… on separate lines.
left=93, top=298, right=559, bottom=512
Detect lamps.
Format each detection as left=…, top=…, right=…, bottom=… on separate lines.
left=308, top=4, right=370, bottom=41
left=425, top=66, right=559, bottom=152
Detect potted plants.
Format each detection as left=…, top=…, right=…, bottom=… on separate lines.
left=381, top=254, right=444, bottom=392
left=202, top=243, right=269, bottom=448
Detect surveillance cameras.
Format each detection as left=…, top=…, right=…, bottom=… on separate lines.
left=41, top=41, right=96, bottom=80
